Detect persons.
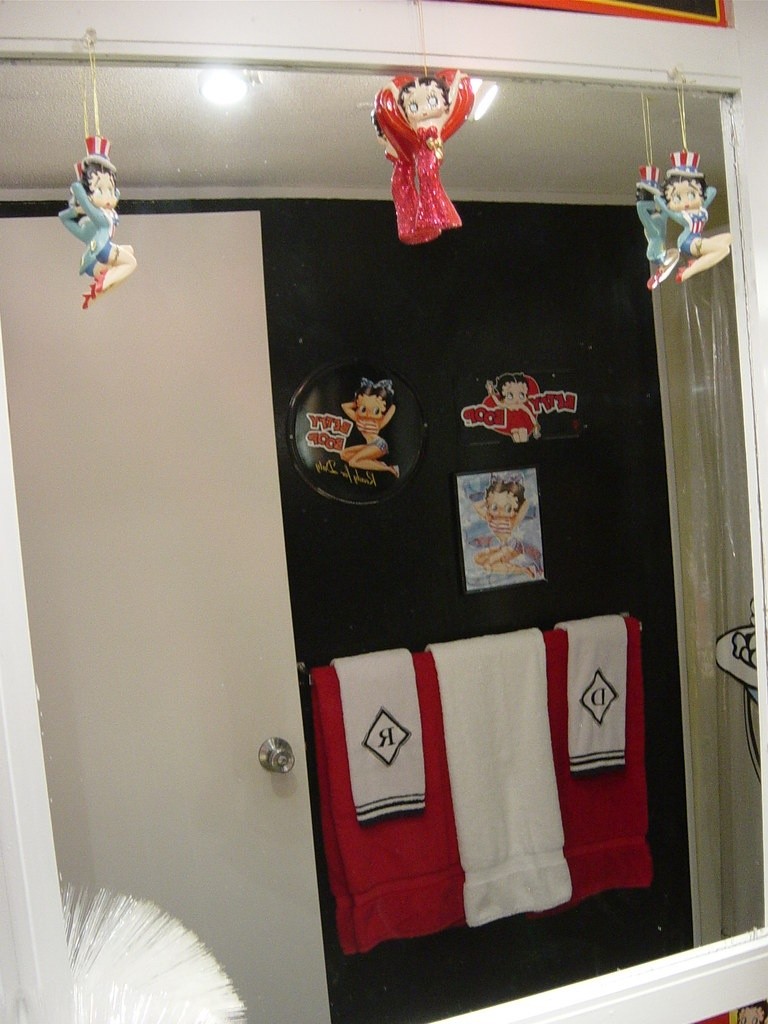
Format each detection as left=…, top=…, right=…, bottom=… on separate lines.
left=60, top=135, right=137, bottom=310
left=636, top=150, right=732, bottom=289
left=370, top=70, right=476, bottom=245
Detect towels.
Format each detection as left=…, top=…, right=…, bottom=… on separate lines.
left=309, top=613, right=655, bottom=956
